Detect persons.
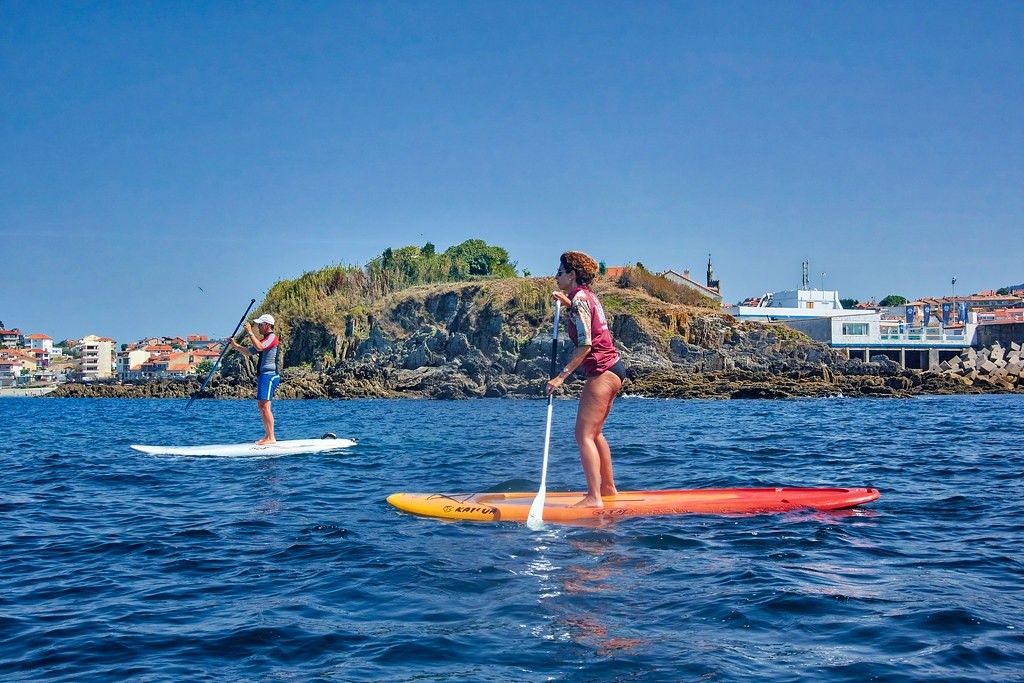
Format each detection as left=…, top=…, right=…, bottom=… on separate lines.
left=545, top=252, right=627, bottom=508
left=227, top=314, right=280, bottom=445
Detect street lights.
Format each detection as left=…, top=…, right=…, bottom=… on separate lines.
left=952, top=277, right=957, bottom=325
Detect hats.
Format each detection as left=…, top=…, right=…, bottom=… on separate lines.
left=253, top=314, right=275, bottom=326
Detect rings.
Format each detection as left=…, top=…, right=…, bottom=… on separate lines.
left=548, top=387, right=550, bottom=390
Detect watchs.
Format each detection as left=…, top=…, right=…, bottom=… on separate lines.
left=248, top=333, right=254, bottom=336
left=563, top=367, right=571, bottom=375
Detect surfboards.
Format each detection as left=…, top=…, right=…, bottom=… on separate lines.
left=385, top=484, right=881, bottom=520
left=128, top=438, right=360, bottom=458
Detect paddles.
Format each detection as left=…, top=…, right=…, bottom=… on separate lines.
left=182, top=297, right=257, bottom=415
left=528, top=298, right=563, bottom=532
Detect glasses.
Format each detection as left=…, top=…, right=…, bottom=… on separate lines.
left=259, top=323, right=267, bottom=326
left=557, top=269, right=567, bottom=276
